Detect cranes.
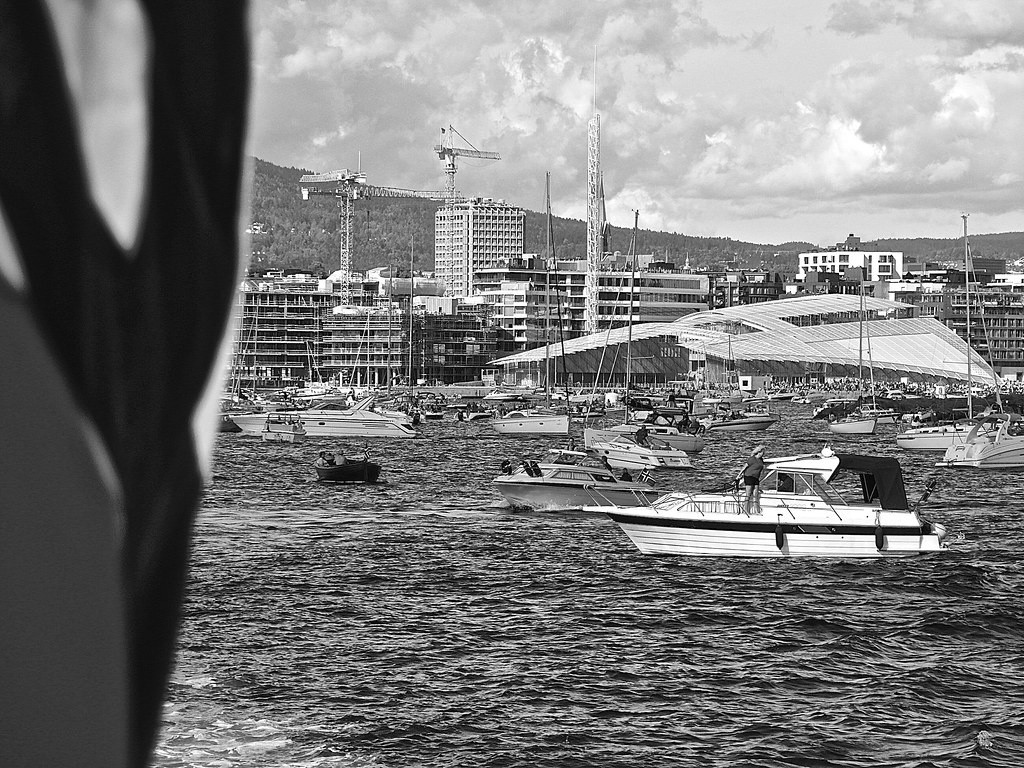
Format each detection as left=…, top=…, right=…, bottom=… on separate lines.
left=432, top=124, right=504, bottom=299
left=296, top=150, right=466, bottom=306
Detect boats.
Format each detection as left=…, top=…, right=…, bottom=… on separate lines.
left=312, top=439, right=383, bottom=484
left=931, top=406, right=1023, bottom=472
left=579, top=437, right=963, bottom=558
left=261, top=409, right=307, bottom=443
left=588, top=426, right=695, bottom=472
left=488, top=435, right=662, bottom=513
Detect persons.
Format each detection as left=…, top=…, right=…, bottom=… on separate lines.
left=620, top=468, right=632, bottom=482
left=563, top=437, right=578, bottom=451
left=769, top=473, right=795, bottom=492
left=731, top=445, right=765, bottom=513
left=634, top=425, right=648, bottom=446
left=333, top=450, right=349, bottom=466
left=316, top=451, right=330, bottom=469
left=601, top=456, right=612, bottom=474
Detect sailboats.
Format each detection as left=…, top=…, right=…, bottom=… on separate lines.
left=211, top=168, right=1024, bottom=455
